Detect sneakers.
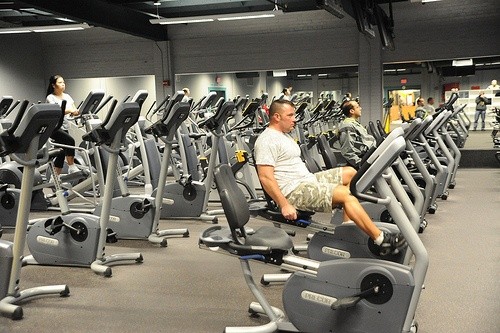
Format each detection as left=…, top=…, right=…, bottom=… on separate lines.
left=375, top=233, right=406, bottom=258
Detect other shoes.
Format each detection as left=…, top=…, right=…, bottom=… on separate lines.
left=49, top=176, right=70, bottom=186
left=68, top=165, right=80, bottom=175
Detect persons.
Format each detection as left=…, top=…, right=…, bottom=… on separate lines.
left=345, top=92, right=352, bottom=101
left=182, top=88, right=190, bottom=103
left=280, top=88, right=291, bottom=101
left=45, top=75, right=82, bottom=183
left=414, top=78, right=500, bottom=132
left=254, top=99, right=408, bottom=258
left=338, top=99, right=408, bottom=194
